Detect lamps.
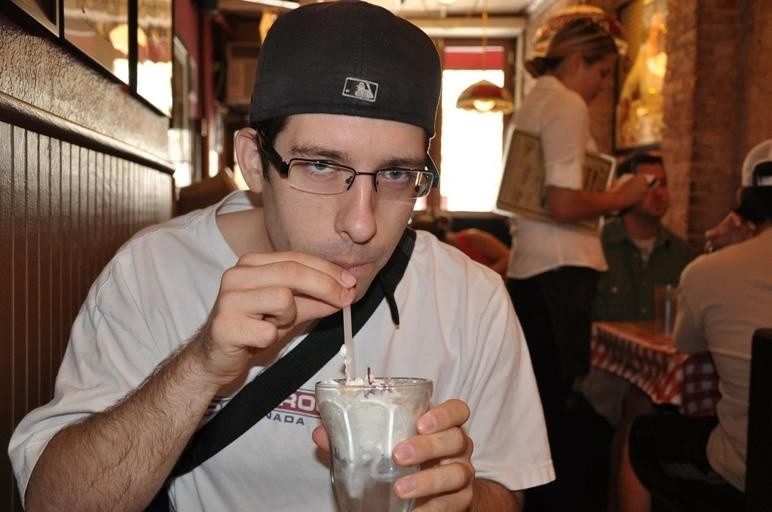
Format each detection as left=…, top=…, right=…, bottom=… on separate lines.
left=459, top=1, right=513, bottom=113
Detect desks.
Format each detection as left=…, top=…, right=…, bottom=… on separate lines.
left=588, top=321, right=718, bottom=511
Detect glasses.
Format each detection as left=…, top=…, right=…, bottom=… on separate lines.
left=257, top=131, right=439, bottom=200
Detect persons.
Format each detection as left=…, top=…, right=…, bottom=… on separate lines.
left=583, top=155, right=692, bottom=511
left=503, top=14, right=650, bottom=510
left=1, top=1, right=558, bottom=511
left=626, top=136, right=772, bottom=511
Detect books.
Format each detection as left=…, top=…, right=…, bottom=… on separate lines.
left=607, top=172, right=657, bottom=216
left=497, top=127, right=616, bottom=238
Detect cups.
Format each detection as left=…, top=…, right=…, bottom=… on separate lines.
left=654, top=285, right=677, bottom=337
left=313, top=375, right=437, bottom=512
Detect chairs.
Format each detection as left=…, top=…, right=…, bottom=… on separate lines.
left=743, top=328, right=771, bottom=512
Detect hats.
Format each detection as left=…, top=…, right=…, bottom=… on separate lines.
left=247, top=0, right=442, bottom=140
left=740, top=139, right=771, bottom=186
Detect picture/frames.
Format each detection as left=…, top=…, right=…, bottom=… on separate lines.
left=60, top=0, right=130, bottom=87
left=0, top=0, right=61, bottom=43
left=130, top=0, right=174, bottom=120
